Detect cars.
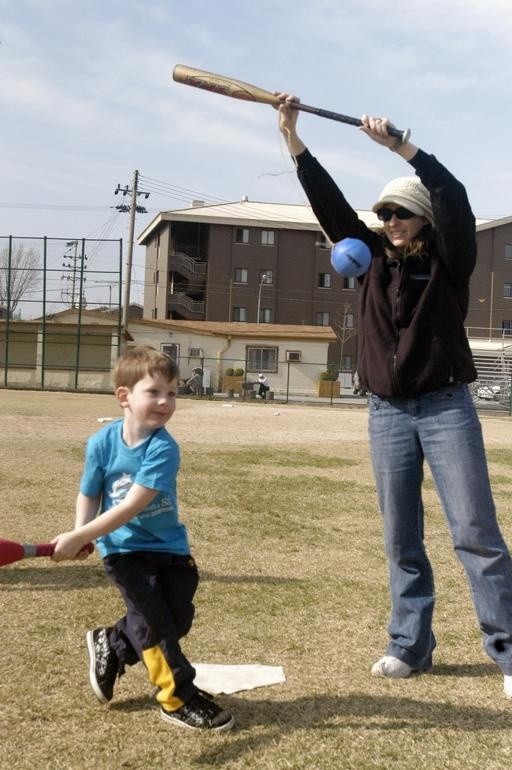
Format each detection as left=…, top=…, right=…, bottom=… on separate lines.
left=477, top=379, right=512, bottom=401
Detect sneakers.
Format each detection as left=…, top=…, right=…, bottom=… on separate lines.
left=159, top=688, right=235, bottom=734
left=371, top=655, right=432, bottom=679
left=87, top=627, right=118, bottom=705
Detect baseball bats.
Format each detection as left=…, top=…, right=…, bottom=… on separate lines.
left=173, top=64, right=410, bottom=144
left=0, top=539, right=94, bottom=566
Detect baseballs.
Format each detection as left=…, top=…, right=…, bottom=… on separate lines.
left=330, top=238, right=371, bottom=277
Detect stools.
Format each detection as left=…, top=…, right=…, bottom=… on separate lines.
left=194, top=386, right=274, bottom=401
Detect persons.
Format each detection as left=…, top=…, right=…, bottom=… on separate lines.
left=268, top=88, right=512, bottom=701
left=188, top=371, right=202, bottom=396
left=48, top=344, right=237, bottom=734
left=256, top=375, right=271, bottom=400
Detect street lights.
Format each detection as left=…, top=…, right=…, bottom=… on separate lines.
left=257, top=274, right=267, bottom=324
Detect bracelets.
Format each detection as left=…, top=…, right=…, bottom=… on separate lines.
left=389, top=136, right=401, bottom=152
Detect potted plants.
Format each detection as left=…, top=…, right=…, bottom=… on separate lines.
left=315, top=370, right=340, bottom=398
left=221, top=368, right=244, bottom=393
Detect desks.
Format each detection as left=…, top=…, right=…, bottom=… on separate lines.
left=236, top=380, right=261, bottom=399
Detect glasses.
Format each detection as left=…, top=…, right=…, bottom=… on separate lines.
left=376, top=207, right=416, bottom=220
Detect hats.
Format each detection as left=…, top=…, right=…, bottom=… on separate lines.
left=373, top=177, right=436, bottom=228
